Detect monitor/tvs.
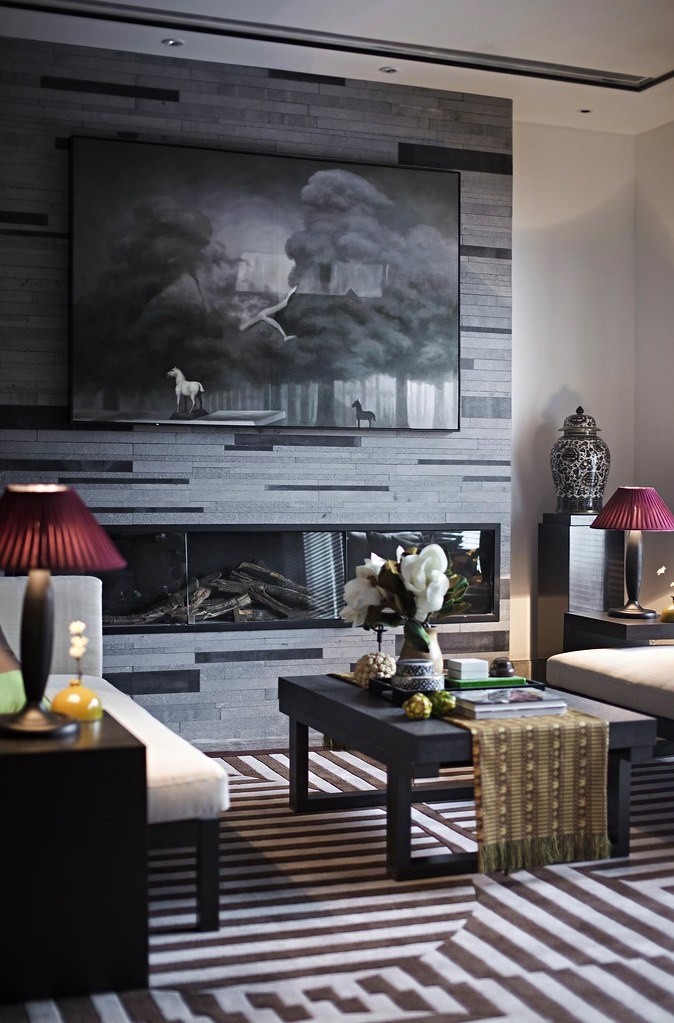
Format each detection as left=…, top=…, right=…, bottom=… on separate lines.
left=65, top=134, right=461, bottom=434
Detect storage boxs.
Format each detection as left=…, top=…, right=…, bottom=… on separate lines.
left=448, top=658, right=489, bottom=680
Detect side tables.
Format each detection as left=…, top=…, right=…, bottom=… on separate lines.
left=560, top=610, right=674, bottom=656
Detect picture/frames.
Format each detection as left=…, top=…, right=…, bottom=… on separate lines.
left=66, top=132, right=463, bottom=431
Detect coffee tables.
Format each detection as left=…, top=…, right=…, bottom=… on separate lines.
left=277, top=672, right=656, bottom=879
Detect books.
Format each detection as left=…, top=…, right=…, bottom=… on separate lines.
left=452, top=687, right=568, bottom=719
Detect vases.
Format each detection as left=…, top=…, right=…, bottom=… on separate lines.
left=660, top=596, right=674, bottom=622
left=400, top=625, right=445, bottom=673
left=550, top=406, right=612, bottom=515
left=53, top=679, right=102, bottom=721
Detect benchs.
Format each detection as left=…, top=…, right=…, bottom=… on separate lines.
left=545, top=644, right=674, bottom=720
left=43, top=673, right=229, bottom=933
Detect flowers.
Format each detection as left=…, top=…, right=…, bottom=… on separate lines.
left=656, top=565, right=674, bottom=587
left=68, top=620, right=89, bottom=681
left=339, top=543, right=472, bottom=652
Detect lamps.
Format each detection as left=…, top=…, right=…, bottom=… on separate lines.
left=0, top=482, right=129, bottom=739
left=589, top=485, right=674, bottom=619
